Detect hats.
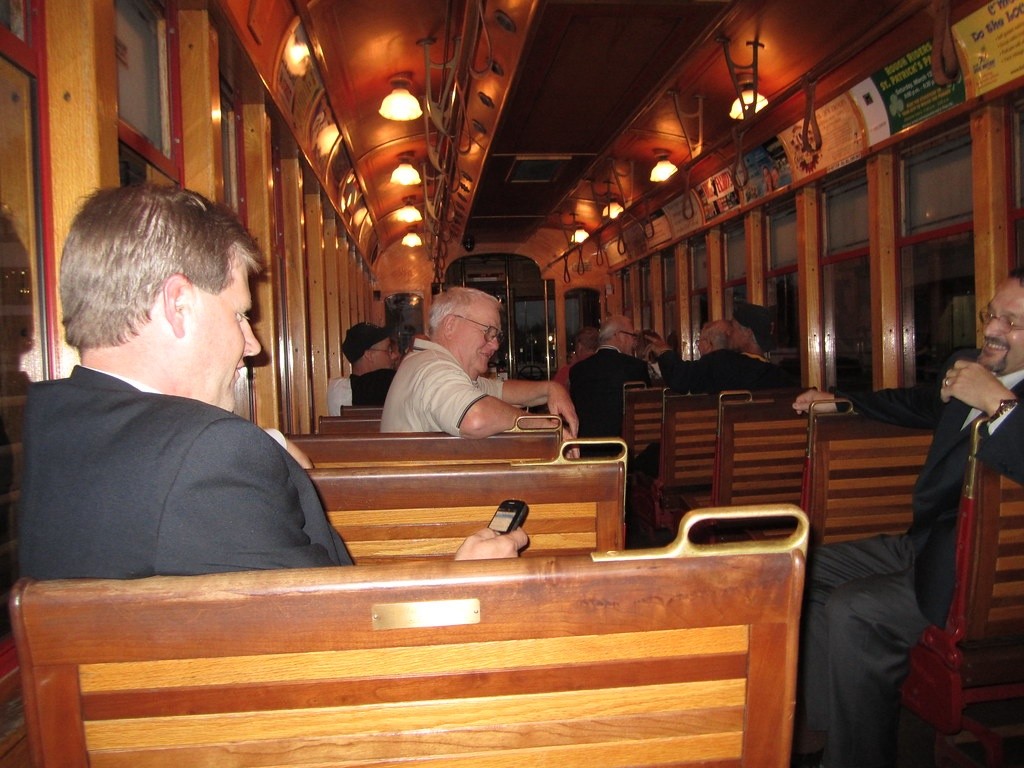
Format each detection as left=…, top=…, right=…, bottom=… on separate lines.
left=342, top=323, right=389, bottom=363
left=733, top=303, right=773, bottom=351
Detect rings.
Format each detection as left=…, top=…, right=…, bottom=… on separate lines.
left=945, top=377, right=949, bottom=387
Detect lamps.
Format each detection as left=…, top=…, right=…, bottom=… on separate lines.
left=391, top=155, right=421, bottom=185
left=402, top=227, right=424, bottom=247
left=731, top=69, right=768, bottom=120
left=603, top=199, right=626, bottom=218
left=395, top=197, right=424, bottom=222
left=650, top=153, right=678, bottom=182
left=379, top=72, right=423, bottom=122
left=570, top=225, right=593, bottom=243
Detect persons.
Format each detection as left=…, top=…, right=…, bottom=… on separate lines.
left=337, top=316, right=402, bottom=407
left=550, top=302, right=788, bottom=493
left=786, top=252, right=1023, bottom=768
left=13, top=182, right=532, bottom=585
left=380, top=283, right=579, bottom=465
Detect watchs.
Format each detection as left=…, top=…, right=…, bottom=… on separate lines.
left=987, top=399, right=1017, bottom=423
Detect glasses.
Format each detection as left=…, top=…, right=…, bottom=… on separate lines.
left=455, top=315, right=504, bottom=341
left=370, top=348, right=393, bottom=357
left=979, top=310, right=1024, bottom=334
left=620, top=330, right=638, bottom=340
left=696, top=339, right=705, bottom=345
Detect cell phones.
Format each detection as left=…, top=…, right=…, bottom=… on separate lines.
left=488, top=500, right=529, bottom=535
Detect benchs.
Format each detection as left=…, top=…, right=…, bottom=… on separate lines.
left=0, top=351, right=1022, bottom=768
